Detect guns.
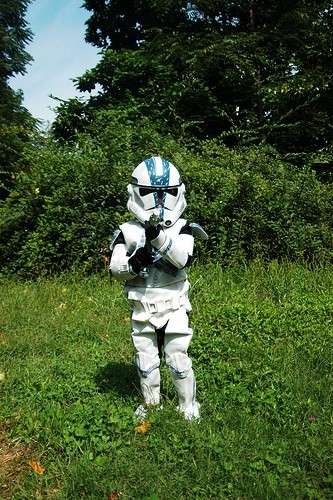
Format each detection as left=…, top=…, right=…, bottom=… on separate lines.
left=138, top=209, right=161, bottom=278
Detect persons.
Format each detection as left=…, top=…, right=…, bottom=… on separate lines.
left=110, top=156, right=209, bottom=422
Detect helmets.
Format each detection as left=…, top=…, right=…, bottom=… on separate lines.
left=125, top=157, right=187, bottom=228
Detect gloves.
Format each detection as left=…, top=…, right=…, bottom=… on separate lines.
left=127, top=247, right=155, bottom=275
left=140, top=220, right=163, bottom=251
